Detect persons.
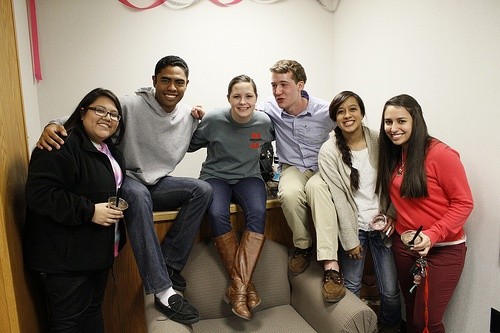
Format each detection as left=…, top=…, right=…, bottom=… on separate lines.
left=21, top=87, right=128, bottom=332
left=187, top=73, right=279, bottom=322
left=32, top=54, right=214, bottom=326
left=373, top=93, right=475, bottom=332
left=315, top=88, right=404, bottom=332
left=190, top=58, right=347, bottom=303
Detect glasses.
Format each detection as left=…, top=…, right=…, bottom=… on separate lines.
left=85, top=106, right=121, bottom=122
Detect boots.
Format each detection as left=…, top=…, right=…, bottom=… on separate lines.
left=223, top=232, right=265, bottom=320
left=212, top=229, right=261, bottom=310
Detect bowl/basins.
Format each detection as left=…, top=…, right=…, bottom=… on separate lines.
left=401, top=230, right=422, bottom=252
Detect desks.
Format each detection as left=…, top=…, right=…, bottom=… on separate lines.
left=101, top=194, right=317, bottom=333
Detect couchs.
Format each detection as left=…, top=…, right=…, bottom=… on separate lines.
left=142, top=237, right=379, bottom=333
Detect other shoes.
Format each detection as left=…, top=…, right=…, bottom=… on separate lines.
left=166, top=265, right=187, bottom=291
left=154, top=292, right=200, bottom=323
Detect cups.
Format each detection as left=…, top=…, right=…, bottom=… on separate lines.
left=108, top=197, right=129, bottom=211
left=370, top=214, right=387, bottom=230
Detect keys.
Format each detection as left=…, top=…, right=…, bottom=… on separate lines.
left=409, top=254, right=429, bottom=293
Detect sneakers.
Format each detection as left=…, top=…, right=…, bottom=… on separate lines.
left=322, top=268, right=346, bottom=303
left=289, top=248, right=311, bottom=273
